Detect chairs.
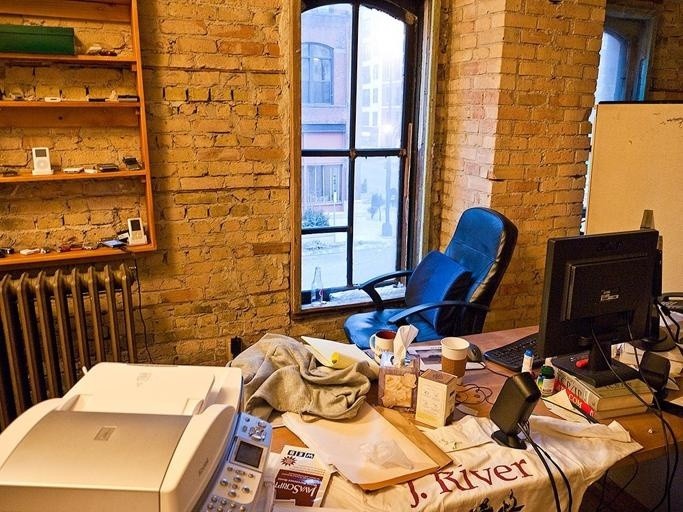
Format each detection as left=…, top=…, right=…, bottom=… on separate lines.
left=341, top=204, right=519, bottom=349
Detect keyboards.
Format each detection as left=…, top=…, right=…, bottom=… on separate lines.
left=483, top=332, right=545, bottom=370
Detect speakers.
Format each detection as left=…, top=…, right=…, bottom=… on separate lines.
left=490, top=372, right=540, bottom=448
left=640, top=352, right=670, bottom=397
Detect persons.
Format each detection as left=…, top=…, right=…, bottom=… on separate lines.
left=360, top=177, right=368, bottom=200
left=370, top=187, right=384, bottom=222
left=390, top=188, right=397, bottom=207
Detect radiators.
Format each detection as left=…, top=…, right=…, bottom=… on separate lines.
left=1, top=261, right=139, bottom=418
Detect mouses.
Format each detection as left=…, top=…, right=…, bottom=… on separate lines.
left=467, top=343, right=484, bottom=362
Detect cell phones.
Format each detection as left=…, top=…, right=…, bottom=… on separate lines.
left=102, top=240, right=126, bottom=248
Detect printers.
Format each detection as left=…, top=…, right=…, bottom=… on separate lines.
left=1, top=359, right=274, bottom=512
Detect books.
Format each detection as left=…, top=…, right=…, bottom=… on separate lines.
left=546, top=356, right=657, bottom=421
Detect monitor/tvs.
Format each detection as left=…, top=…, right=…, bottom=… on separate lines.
left=538, top=228, right=659, bottom=388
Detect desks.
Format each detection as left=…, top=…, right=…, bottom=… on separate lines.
left=1, top=324, right=682, bottom=512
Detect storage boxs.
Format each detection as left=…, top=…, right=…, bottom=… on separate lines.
left=0, top=24, right=74, bottom=55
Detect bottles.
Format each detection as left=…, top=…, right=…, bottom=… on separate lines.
left=534, top=366, right=554, bottom=397
left=522, top=350, right=534, bottom=376
left=311, top=267, right=323, bottom=305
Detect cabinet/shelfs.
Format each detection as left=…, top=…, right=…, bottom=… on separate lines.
left=0, top=1, right=157, bottom=267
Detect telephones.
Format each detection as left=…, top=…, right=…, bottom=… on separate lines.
left=660, top=292, right=683, bottom=314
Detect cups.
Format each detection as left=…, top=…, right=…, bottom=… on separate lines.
left=368, top=330, right=395, bottom=364
left=440, top=337, right=470, bottom=385
